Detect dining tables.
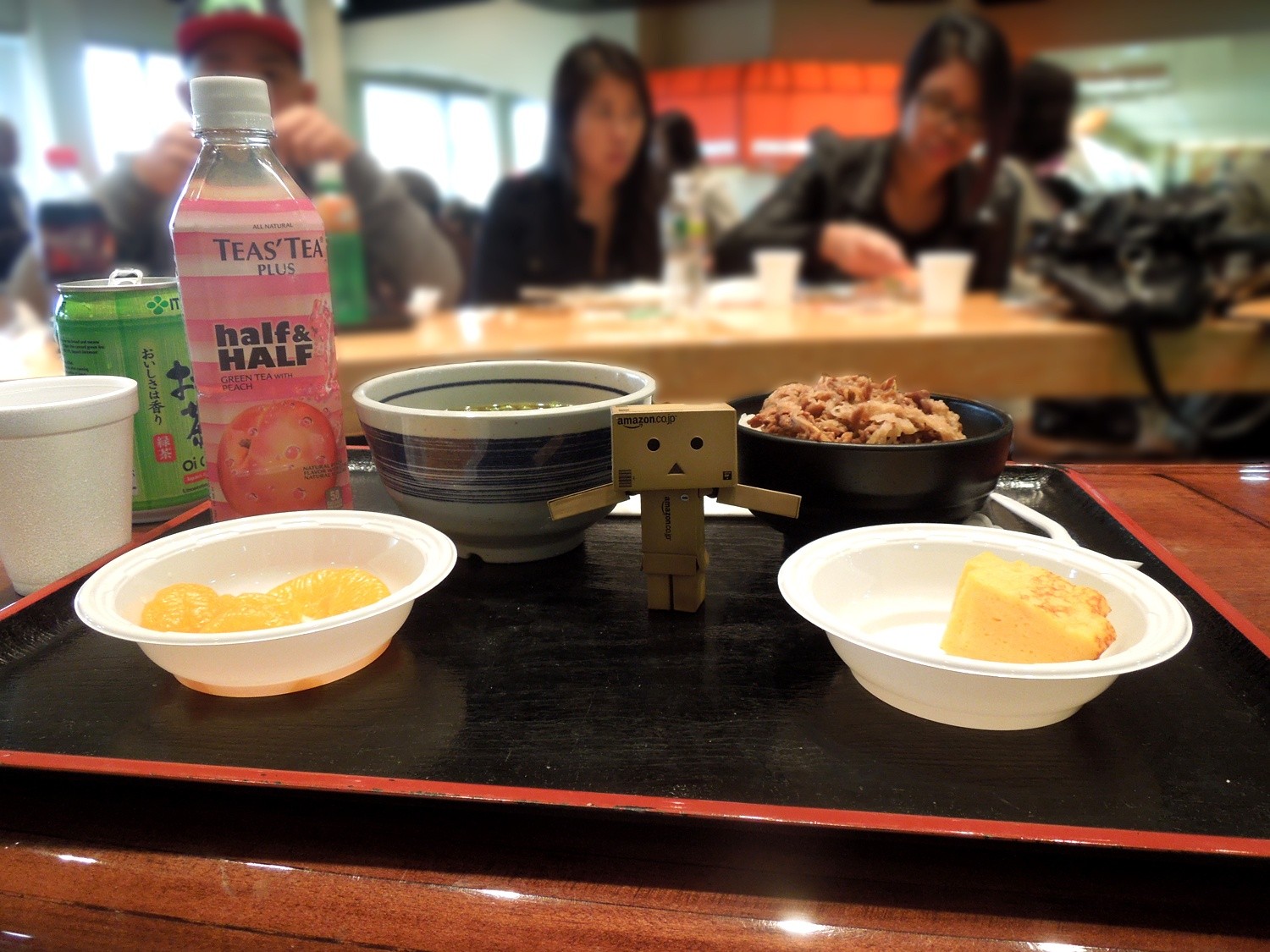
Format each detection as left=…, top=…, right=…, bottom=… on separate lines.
left=335, top=274, right=1270, bottom=438
left=0, top=460, right=1270, bottom=952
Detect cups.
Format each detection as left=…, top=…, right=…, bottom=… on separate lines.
left=754, top=247, right=803, bottom=307
left=0, top=373, right=141, bottom=596
left=919, top=249, right=977, bottom=318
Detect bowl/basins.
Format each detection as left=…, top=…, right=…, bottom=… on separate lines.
left=68, top=360, right=1198, bottom=732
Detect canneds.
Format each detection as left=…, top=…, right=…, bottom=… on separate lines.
left=54, top=266, right=209, bottom=523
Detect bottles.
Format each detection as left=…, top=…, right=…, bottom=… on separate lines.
left=658, top=174, right=713, bottom=317
left=53, top=76, right=375, bottom=524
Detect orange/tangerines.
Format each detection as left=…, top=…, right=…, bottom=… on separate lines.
left=141, top=566, right=390, bottom=636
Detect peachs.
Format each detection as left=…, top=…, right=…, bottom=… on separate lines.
left=216, top=402, right=340, bottom=517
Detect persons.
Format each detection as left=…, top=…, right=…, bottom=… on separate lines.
left=1, top=117, right=31, bottom=291
left=1007, top=52, right=1093, bottom=210
left=648, top=112, right=745, bottom=285
left=714, top=11, right=1027, bottom=296
left=1, top=0, right=470, bottom=326
left=475, top=35, right=669, bottom=305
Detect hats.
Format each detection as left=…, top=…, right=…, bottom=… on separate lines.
left=177, top=0, right=304, bottom=62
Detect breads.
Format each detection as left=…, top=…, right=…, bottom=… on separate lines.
left=938, top=552, right=1118, bottom=665
